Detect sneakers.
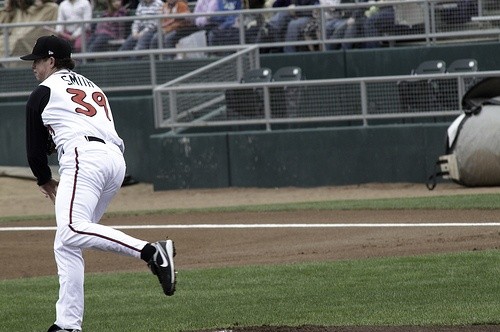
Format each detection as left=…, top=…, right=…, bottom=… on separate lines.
left=148, top=239, right=177, bottom=295
left=47, top=325, right=81, bottom=332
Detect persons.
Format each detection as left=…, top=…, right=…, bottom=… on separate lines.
left=177, top=0, right=395, bottom=57
left=20, top=35, right=176, bottom=332
left=0, top=0, right=191, bottom=67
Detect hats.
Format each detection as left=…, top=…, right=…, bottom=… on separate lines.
left=20, top=35, right=72, bottom=61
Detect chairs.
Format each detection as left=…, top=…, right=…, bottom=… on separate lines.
left=239, top=58, right=477, bottom=83
